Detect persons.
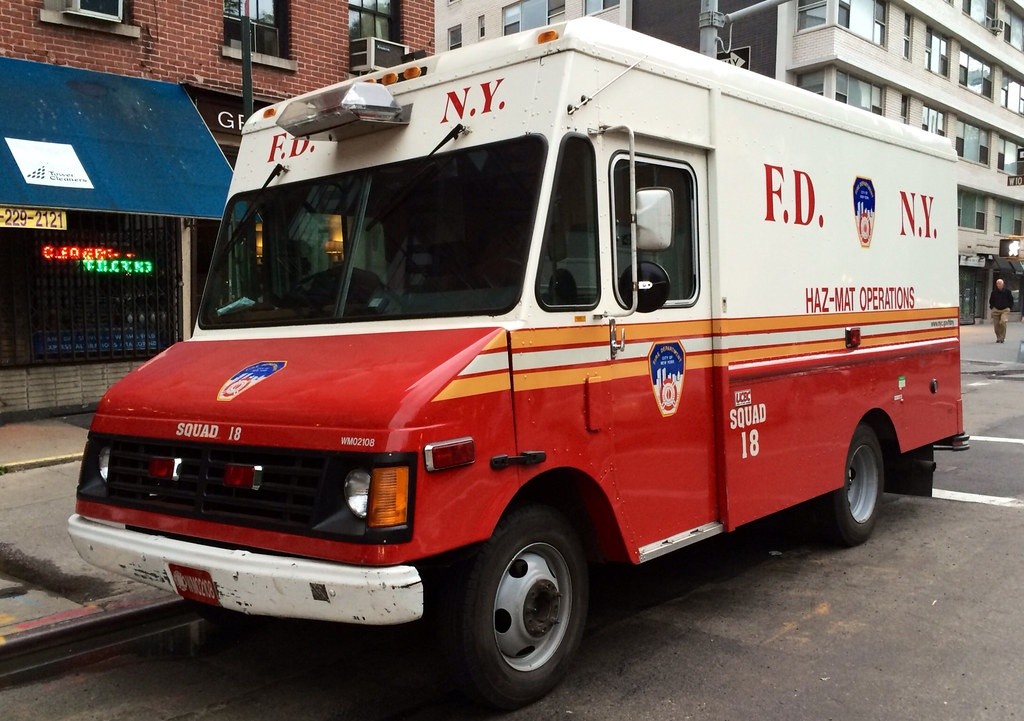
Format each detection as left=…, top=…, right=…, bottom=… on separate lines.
left=989, top=278, right=1014, bottom=343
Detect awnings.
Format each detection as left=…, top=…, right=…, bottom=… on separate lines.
left=0, top=57, right=264, bottom=222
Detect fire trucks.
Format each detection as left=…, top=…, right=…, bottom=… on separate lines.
left=67, top=16, right=970, bottom=713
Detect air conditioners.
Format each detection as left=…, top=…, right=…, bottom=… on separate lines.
left=62, top=0, right=123, bottom=22
left=349, top=36, right=409, bottom=72
